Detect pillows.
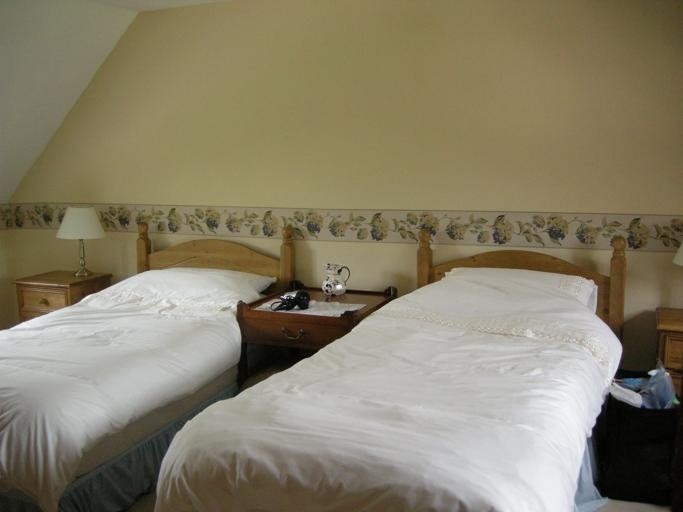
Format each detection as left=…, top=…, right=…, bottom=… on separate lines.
left=443, top=266, right=599, bottom=315
left=154, top=266, right=275, bottom=304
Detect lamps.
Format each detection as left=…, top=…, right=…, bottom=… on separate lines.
left=56, top=208, right=103, bottom=278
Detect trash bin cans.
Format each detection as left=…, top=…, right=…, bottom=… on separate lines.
left=600, top=370, right=682, bottom=505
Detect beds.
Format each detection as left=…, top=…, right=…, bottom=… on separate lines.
left=149, top=226, right=623, bottom=511
left=1, top=220, right=292, bottom=512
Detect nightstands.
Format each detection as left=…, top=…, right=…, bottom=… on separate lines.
left=235, top=284, right=398, bottom=396
left=653, top=308, right=683, bottom=400
